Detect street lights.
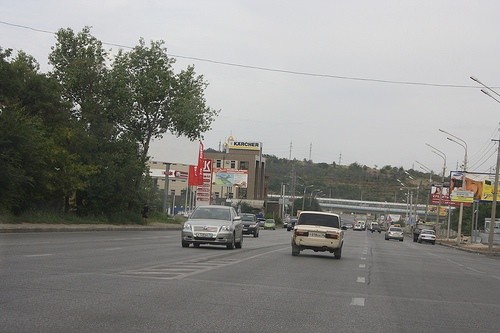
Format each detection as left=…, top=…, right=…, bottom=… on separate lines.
left=396, top=128, right=468, bottom=246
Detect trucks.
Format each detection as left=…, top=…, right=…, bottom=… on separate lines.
left=410, top=223, right=436, bottom=243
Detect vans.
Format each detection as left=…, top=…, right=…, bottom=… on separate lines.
left=291, top=210, right=348, bottom=259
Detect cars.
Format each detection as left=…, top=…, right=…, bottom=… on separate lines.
left=385, top=224, right=404, bottom=242
left=340, top=217, right=391, bottom=234
left=287, top=219, right=298, bottom=231
left=417, top=229, right=436, bottom=246
left=283, top=222, right=288, bottom=228
left=256, top=218, right=266, bottom=228
left=263, top=219, right=276, bottom=230
left=237, top=213, right=260, bottom=237
left=180, top=204, right=244, bottom=250
